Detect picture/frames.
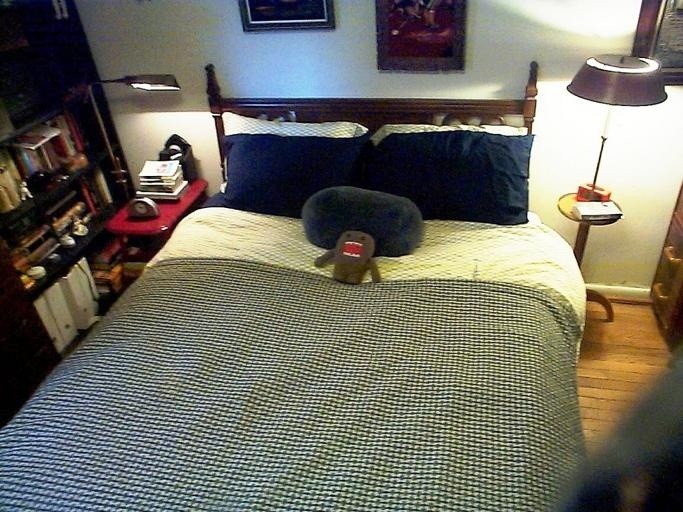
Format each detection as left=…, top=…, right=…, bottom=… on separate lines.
left=375, top=1, right=467, bottom=73
left=237, top=0, right=336, bottom=33
left=630, top=0, right=683, bottom=85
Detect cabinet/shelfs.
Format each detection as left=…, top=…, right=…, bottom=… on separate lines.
left=0, top=1, right=148, bottom=397
left=650, top=181, right=682, bottom=350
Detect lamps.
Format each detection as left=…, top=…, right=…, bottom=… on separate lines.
left=556, top=53, right=667, bottom=323
left=87, top=74, right=182, bottom=206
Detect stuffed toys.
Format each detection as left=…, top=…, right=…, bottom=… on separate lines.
left=313, top=228, right=380, bottom=285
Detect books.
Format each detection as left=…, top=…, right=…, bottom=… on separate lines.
left=0, top=112, right=123, bottom=300
left=571, top=201, right=623, bottom=221
left=132, top=158, right=188, bottom=202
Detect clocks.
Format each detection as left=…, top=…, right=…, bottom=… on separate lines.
left=126, top=195, right=159, bottom=220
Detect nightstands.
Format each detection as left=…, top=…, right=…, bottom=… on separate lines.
left=104, top=174, right=210, bottom=293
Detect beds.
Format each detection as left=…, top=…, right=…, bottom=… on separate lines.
left=1, top=62, right=587, bottom=512
